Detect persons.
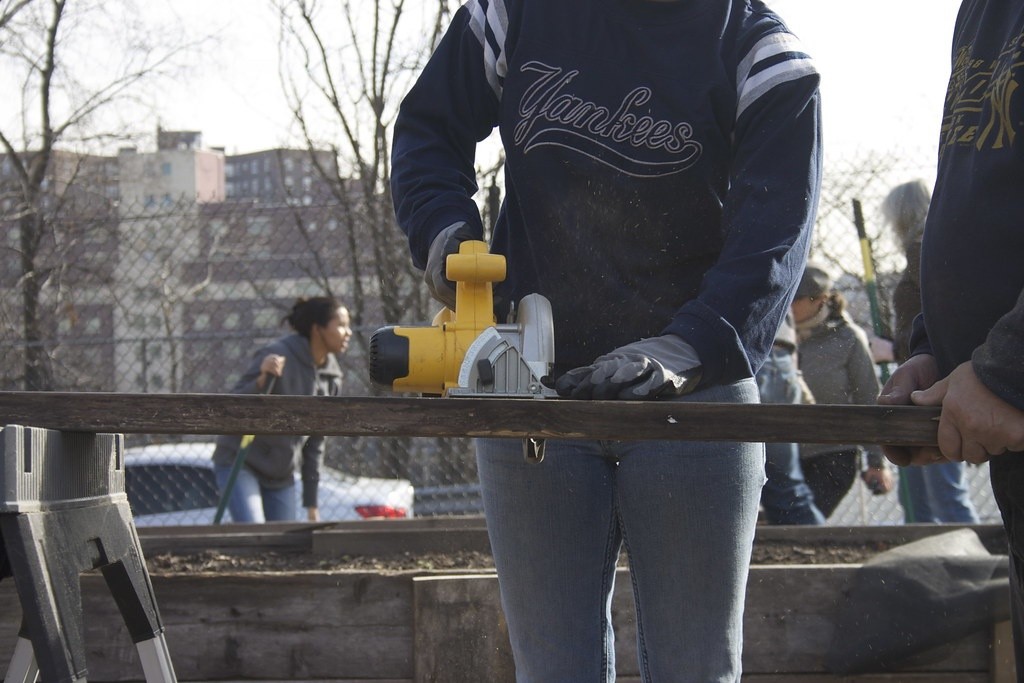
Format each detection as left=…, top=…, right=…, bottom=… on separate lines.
left=212, top=296, right=354, bottom=525
left=874, top=0, right=1024, bottom=683
left=393, top=1, right=824, bottom=682
left=756, top=306, right=822, bottom=525
left=870, top=179, right=979, bottom=523
left=791, top=265, right=890, bottom=519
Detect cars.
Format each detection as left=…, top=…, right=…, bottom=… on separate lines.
left=121, top=442, right=414, bottom=529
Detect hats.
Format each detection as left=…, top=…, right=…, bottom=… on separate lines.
left=793, top=266, right=829, bottom=301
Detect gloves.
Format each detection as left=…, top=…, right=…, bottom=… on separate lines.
left=539, top=333, right=703, bottom=400
left=424, top=221, right=502, bottom=312
